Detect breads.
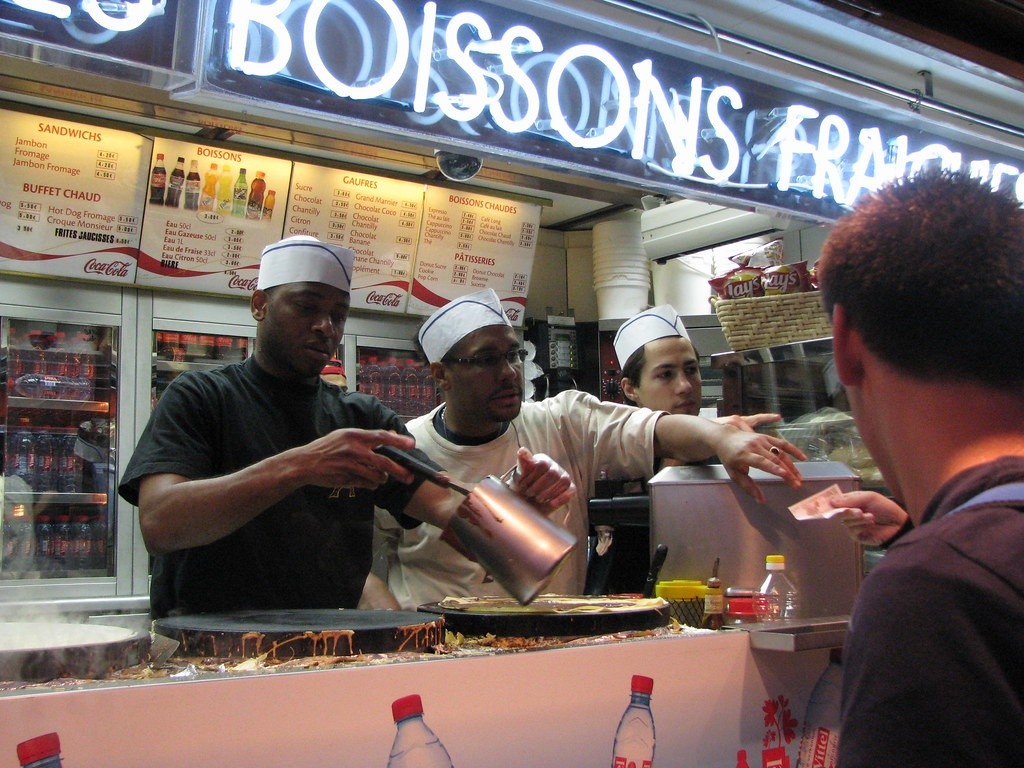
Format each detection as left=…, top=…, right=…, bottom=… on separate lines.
left=763, top=405, right=886, bottom=484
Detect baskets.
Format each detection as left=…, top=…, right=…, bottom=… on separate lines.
left=707, top=291, right=834, bottom=351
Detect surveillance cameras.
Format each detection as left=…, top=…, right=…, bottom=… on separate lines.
left=434, top=148, right=483, bottom=181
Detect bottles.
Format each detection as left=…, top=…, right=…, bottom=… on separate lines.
left=612, top=675, right=655, bottom=768
left=701, top=575, right=724, bottom=632
left=386, top=694, right=457, bottom=768
left=726, top=599, right=778, bottom=624
left=0, top=330, right=437, bottom=571
left=753, top=554, right=799, bottom=623
left=16, top=731, right=67, bottom=768
left=152, top=152, right=276, bottom=222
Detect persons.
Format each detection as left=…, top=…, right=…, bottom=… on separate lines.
left=810, top=168, right=1024, bottom=768
left=831, top=490, right=916, bottom=548
left=112, top=236, right=579, bottom=625
left=583, top=305, right=725, bottom=594
left=359, top=288, right=807, bottom=609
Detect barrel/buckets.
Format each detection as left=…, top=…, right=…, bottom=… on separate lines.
left=592, top=221, right=651, bottom=320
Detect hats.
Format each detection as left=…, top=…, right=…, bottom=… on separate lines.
left=256, top=235, right=354, bottom=294
left=418, top=288, right=514, bottom=364
left=613, top=304, right=691, bottom=370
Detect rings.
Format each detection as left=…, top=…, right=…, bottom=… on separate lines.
left=770, top=446, right=779, bottom=455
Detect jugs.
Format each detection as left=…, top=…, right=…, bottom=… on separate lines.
left=440, top=462, right=580, bottom=608
